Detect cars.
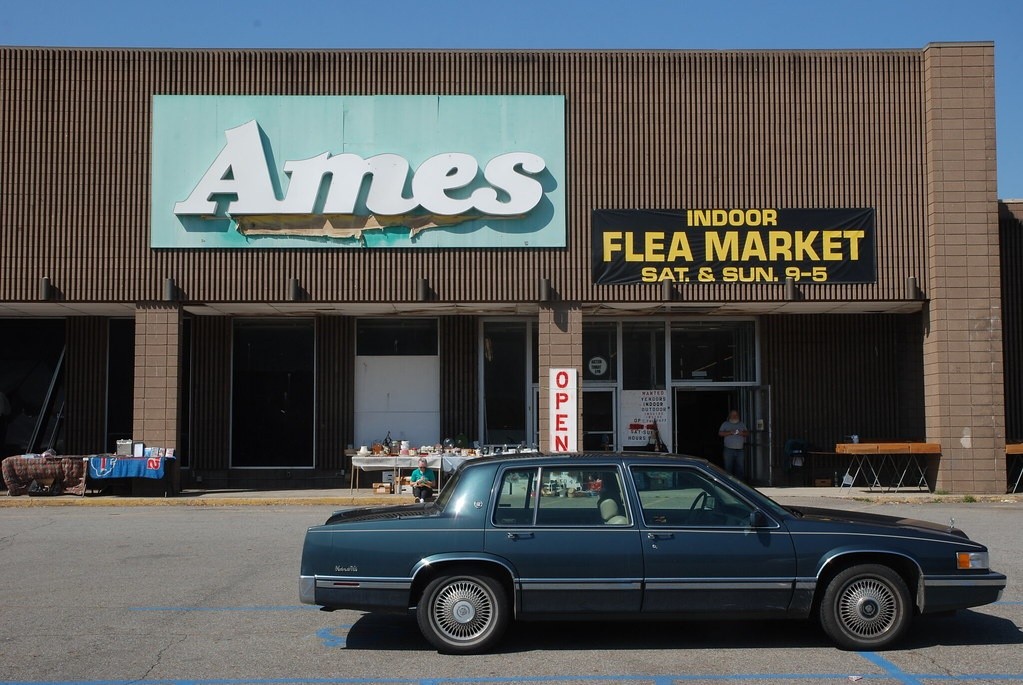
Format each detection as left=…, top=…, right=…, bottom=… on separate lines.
left=298, top=452, right=1008, bottom=654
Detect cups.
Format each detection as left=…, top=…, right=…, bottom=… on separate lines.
left=461, top=449, right=467, bottom=456
left=852, top=435, right=859, bottom=443
left=361, top=446, right=367, bottom=452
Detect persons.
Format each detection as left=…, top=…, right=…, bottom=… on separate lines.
left=410, top=458, right=435, bottom=503
left=719, top=410, right=749, bottom=480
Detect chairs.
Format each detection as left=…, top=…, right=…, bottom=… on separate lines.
left=596, top=497, right=627, bottom=525
left=784, top=438, right=809, bottom=486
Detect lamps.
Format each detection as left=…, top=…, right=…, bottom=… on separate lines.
left=539, top=278, right=551, bottom=302
left=39, top=277, right=50, bottom=302
left=163, top=278, right=175, bottom=301
left=785, top=277, right=795, bottom=301
left=288, top=278, right=299, bottom=302
left=662, top=279, right=672, bottom=301
left=416, top=279, right=428, bottom=303
left=906, top=276, right=916, bottom=300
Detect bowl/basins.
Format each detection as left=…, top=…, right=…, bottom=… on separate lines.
left=356, top=450, right=372, bottom=457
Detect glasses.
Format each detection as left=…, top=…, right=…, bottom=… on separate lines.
left=420, top=467, right=426, bottom=468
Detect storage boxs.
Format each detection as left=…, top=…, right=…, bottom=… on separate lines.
left=815, top=479, right=832, bottom=487
left=371, top=471, right=413, bottom=495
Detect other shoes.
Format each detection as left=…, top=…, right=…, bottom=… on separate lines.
left=415, top=497, right=420, bottom=503
left=419, top=499, right=424, bottom=502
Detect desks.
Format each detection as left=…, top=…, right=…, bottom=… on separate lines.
left=806, top=451, right=838, bottom=487
left=350, top=456, right=478, bottom=497
left=835, top=443, right=940, bottom=494
left=1005, top=443, right=1023, bottom=493
left=80, top=456, right=177, bottom=498
left=2, top=454, right=91, bottom=497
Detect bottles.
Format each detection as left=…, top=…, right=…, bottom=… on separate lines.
left=454, top=432, right=469, bottom=449
left=401, top=441, right=409, bottom=456
left=390, top=441, right=399, bottom=456
left=383, top=430, right=393, bottom=447
